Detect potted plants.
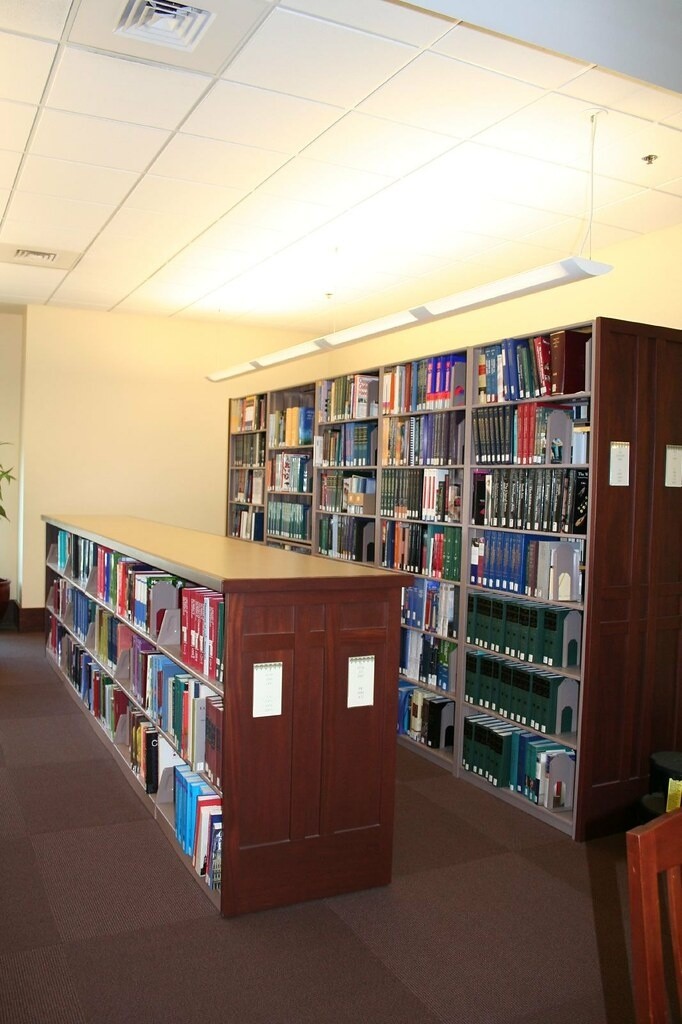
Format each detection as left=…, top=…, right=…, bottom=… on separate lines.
left=0, top=440, right=12, bottom=607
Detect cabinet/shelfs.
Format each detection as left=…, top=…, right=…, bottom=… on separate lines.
left=313, top=365, right=384, bottom=568
left=263, top=382, right=324, bottom=550
left=371, top=348, right=475, bottom=779
left=224, top=393, right=271, bottom=545
left=453, top=318, right=682, bottom=841
left=39, top=513, right=415, bottom=916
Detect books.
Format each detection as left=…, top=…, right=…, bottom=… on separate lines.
left=231, top=330, right=592, bottom=813
left=49, top=530, right=226, bottom=891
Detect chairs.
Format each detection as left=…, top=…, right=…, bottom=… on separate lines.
left=626, top=809, right=682, bottom=1024
